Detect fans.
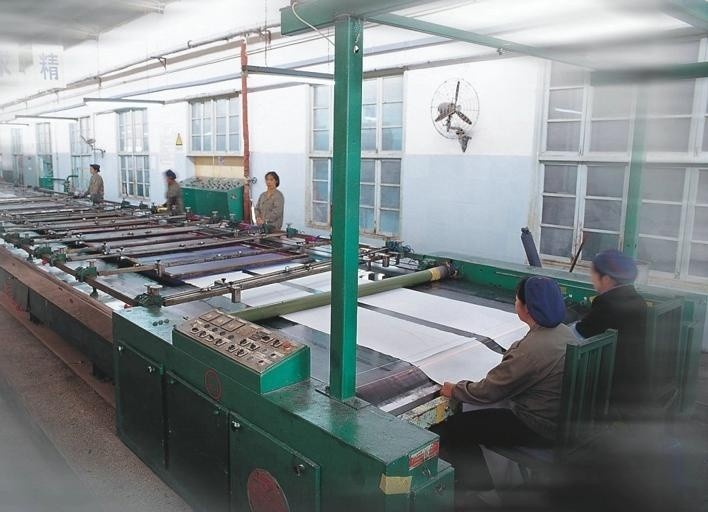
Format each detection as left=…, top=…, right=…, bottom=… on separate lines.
left=429, top=77, right=481, bottom=154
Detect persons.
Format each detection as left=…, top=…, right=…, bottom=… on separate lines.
left=155, top=169, right=184, bottom=213
left=254, top=171, right=285, bottom=232
left=439, top=275, right=582, bottom=511
left=78, top=163, right=104, bottom=201
left=566, top=246, right=652, bottom=414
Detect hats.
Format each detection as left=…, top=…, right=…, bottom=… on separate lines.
left=592, top=249, right=637, bottom=279
left=90, top=164, right=100, bottom=172
left=166, top=169, right=176, bottom=179
left=524, top=275, right=566, bottom=328
left=265, top=172, right=280, bottom=187
